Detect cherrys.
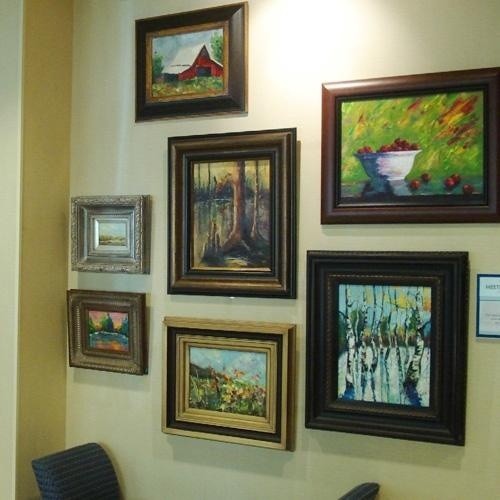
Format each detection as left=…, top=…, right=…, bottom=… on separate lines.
left=357, top=136, right=420, bottom=153
left=410, top=173, right=474, bottom=194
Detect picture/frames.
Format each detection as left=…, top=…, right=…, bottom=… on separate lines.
left=305, top=249, right=468, bottom=445
left=71, top=194, right=149, bottom=274
left=167, top=128, right=296, bottom=300
left=136, top=0, right=249, bottom=122
left=65, top=289, right=146, bottom=374
left=160, top=316, right=297, bottom=452
left=320, top=68, right=500, bottom=225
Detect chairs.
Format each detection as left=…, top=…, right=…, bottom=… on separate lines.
left=31, top=443, right=122, bottom=500
left=337, top=483, right=379, bottom=500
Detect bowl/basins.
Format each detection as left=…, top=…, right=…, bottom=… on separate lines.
left=354, top=149, right=419, bottom=180
